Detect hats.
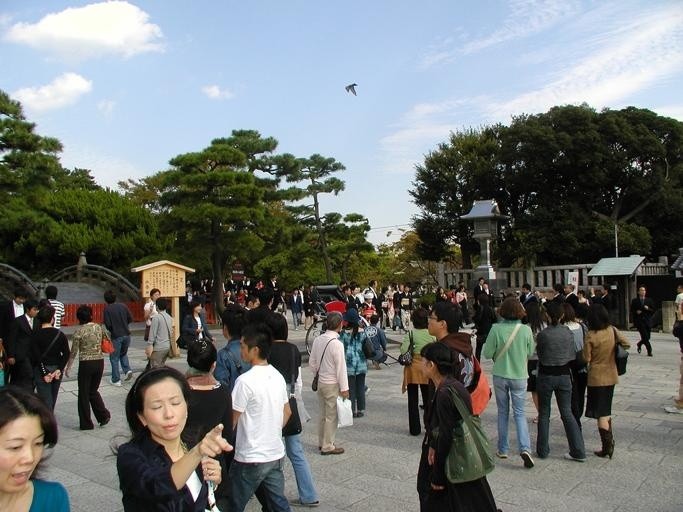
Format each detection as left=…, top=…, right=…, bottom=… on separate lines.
left=343, top=308, right=360, bottom=323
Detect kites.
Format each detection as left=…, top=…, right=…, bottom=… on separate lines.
left=345, top=83, right=359, bottom=96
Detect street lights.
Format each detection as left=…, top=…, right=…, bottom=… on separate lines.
left=458, top=198, right=513, bottom=280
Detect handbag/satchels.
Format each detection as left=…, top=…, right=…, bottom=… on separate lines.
left=433, top=415, right=495, bottom=483
left=282, top=397, right=301, bottom=436
left=673, top=318, right=683, bottom=336
left=177, top=333, right=199, bottom=350
left=399, top=348, right=413, bottom=365
left=312, top=375, right=319, bottom=391
left=615, top=342, right=628, bottom=376
left=469, top=372, right=491, bottom=415
left=169, top=330, right=180, bottom=356
left=362, top=337, right=376, bottom=358
left=101, top=337, right=115, bottom=354
left=39, top=361, right=59, bottom=375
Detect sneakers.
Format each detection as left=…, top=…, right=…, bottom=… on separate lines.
left=665, top=406, right=683, bottom=413
left=352, top=412, right=364, bottom=418
left=496, top=448, right=587, bottom=468
left=110, top=376, right=122, bottom=388
left=100, top=418, right=110, bottom=426
left=289, top=498, right=320, bottom=506
left=124, top=371, right=133, bottom=382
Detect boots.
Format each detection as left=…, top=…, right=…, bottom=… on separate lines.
left=595, top=419, right=615, bottom=459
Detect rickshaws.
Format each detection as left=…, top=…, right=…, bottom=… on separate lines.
left=305, top=300, right=404, bottom=367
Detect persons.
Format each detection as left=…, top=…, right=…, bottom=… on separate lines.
left=0, top=278, right=683, bottom=512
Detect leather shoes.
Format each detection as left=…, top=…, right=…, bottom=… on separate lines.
left=648, top=351, right=652, bottom=356
left=637, top=343, right=642, bottom=352
left=319, top=444, right=344, bottom=454
